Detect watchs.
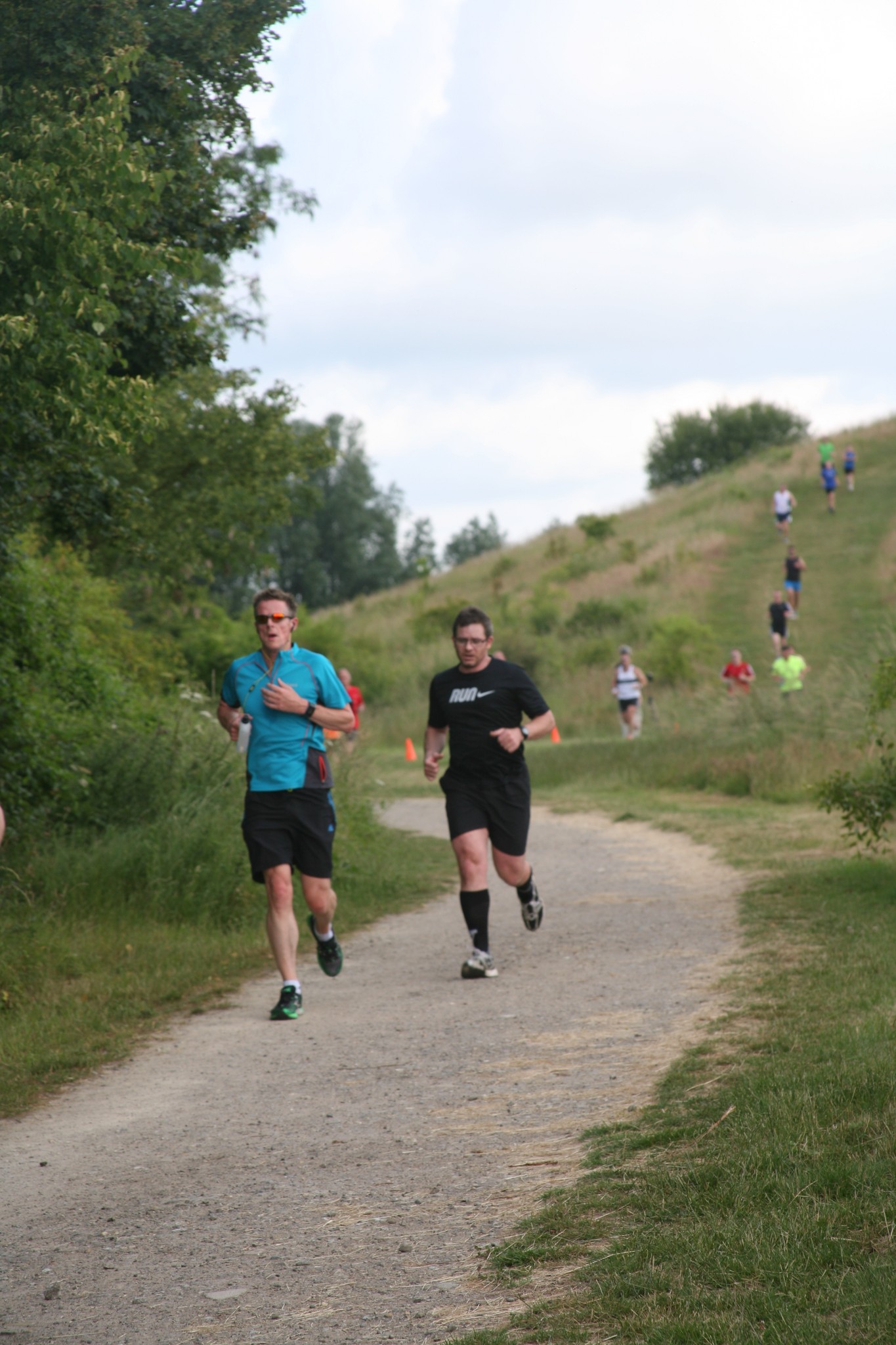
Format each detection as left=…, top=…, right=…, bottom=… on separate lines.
left=305, top=702, right=315, bottom=716
left=520, top=725, right=529, bottom=738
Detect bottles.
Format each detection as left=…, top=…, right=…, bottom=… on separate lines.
left=235, top=715, right=251, bottom=754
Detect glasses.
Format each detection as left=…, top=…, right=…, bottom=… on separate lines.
left=255, top=613, right=293, bottom=624
left=457, top=637, right=489, bottom=645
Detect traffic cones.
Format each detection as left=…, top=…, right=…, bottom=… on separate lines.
left=405, top=738, right=417, bottom=762
left=550, top=726, right=561, bottom=744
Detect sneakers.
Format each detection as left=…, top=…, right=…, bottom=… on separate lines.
left=270, top=985, right=304, bottom=1020
left=517, top=883, right=543, bottom=932
left=461, top=950, right=498, bottom=980
left=307, top=912, right=343, bottom=976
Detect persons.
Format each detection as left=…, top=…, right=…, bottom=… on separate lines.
left=770, top=480, right=798, bottom=543
left=721, top=649, right=756, bottom=695
left=782, top=544, right=807, bottom=620
left=611, top=643, right=649, bottom=739
left=766, top=587, right=811, bottom=704
left=215, top=588, right=365, bottom=1021
left=424, top=604, right=556, bottom=975
left=817, top=435, right=858, bottom=511
